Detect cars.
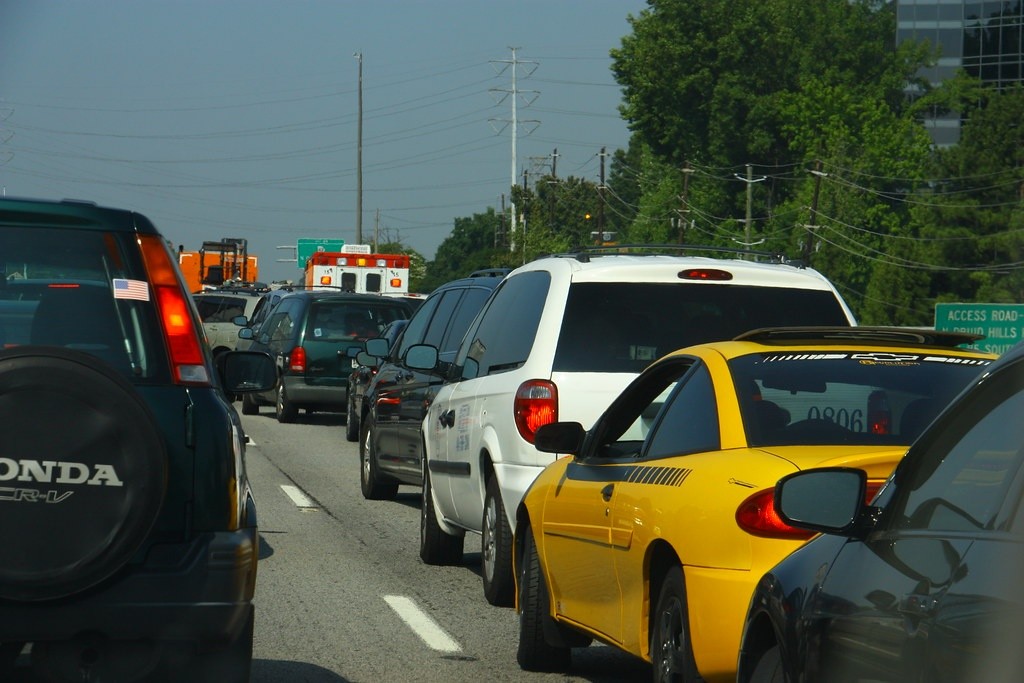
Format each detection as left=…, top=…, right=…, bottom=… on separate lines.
left=733, top=340, right=1022, bottom=683
left=344, top=319, right=407, bottom=442
left=505, top=326, right=1005, bottom=683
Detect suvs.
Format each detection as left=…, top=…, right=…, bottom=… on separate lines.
left=401, top=242, right=860, bottom=606
left=0, top=192, right=259, bottom=683
left=355, top=262, right=515, bottom=501
left=190, top=279, right=426, bottom=421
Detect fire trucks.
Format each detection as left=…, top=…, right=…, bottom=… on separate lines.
left=305, top=243, right=414, bottom=302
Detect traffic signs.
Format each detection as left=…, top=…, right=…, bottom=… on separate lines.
left=296, top=238, right=344, bottom=270
